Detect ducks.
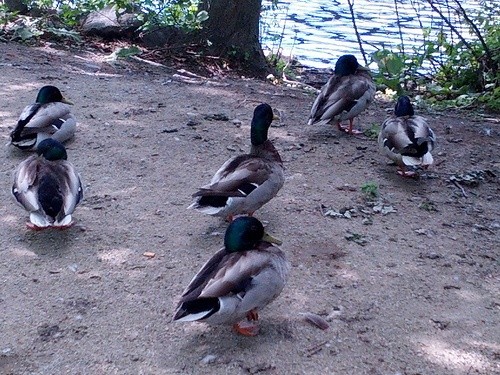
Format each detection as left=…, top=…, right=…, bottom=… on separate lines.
left=378, top=94, right=437, bottom=179
left=307, top=54, right=377, bottom=136
left=171, top=216, right=291, bottom=336
left=10, top=138, right=83, bottom=231
left=5, top=85, right=76, bottom=150
left=186, top=102, right=286, bottom=222
